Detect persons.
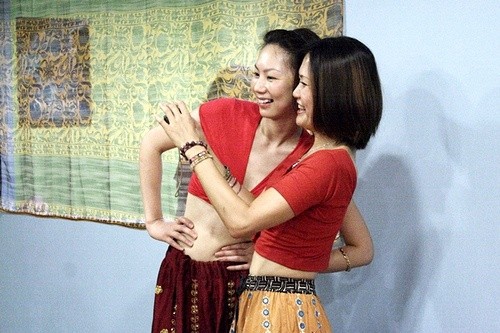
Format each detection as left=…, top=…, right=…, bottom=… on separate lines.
left=155, top=34, right=382, bottom=332
left=137, top=26, right=375, bottom=332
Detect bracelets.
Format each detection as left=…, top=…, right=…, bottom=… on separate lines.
left=339, top=246, right=351, bottom=271
left=180, top=139, right=208, bottom=159
left=223, top=163, right=243, bottom=196
left=187, top=149, right=213, bottom=172
